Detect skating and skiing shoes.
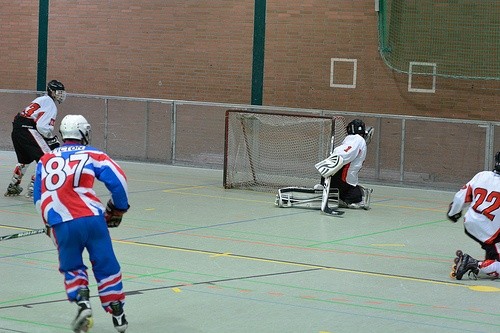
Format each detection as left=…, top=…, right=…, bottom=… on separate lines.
left=450, top=250, right=481, bottom=280
left=72, top=289, right=93, bottom=333
left=109, top=301, right=128, bottom=333
left=26, top=180, right=34, bottom=198
left=4, top=183, right=23, bottom=197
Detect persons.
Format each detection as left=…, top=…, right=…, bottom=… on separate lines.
left=315, top=118, right=374, bottom=209
left=447, top=151, right=500, bottom=280
left=34, top=114, right=131, bottom=333
left=4, top=80, right=66, bottom=198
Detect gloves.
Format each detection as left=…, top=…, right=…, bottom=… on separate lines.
left=103, top=199, right=130, bottom=227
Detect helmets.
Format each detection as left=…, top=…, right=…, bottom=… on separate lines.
left=47, top=80, right=64, bottom=91
left=60, top=115, right=91, bottom=140
left=347, top=119, right=365, bottom=134
